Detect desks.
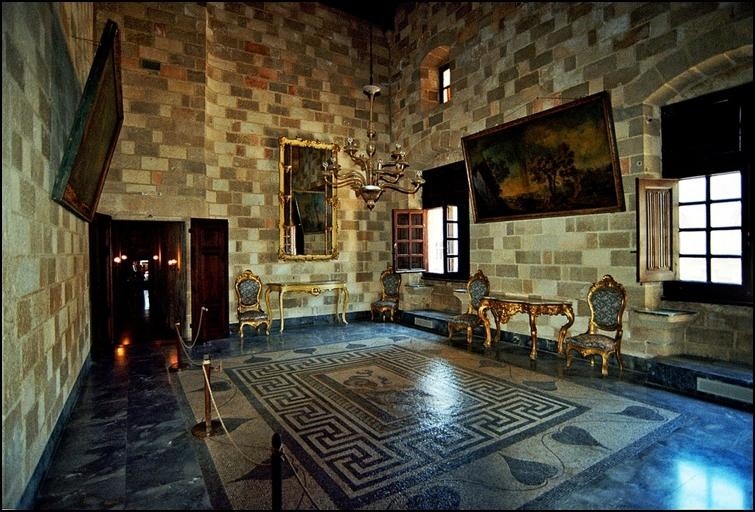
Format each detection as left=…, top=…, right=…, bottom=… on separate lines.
left=266, top=281, right=350, bottom=333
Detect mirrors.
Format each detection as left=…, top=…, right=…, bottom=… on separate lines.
left=279, top=138, right=338, bottom=262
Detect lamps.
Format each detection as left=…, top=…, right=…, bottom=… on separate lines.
left=323, top=13, right=426, bottom=214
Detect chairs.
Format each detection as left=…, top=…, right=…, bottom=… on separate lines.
left=371, top=268, right=403, bottom=323
left=236, top=271, right=272, bottom=338
left=448, top=270, right=491, bottom=344
left=564, top=274, right=628, bottom=376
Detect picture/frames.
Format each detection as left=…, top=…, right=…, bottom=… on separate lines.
left=52, top=19, right=125, bottom=224
left=462, top=91, right=626, bottom=225
